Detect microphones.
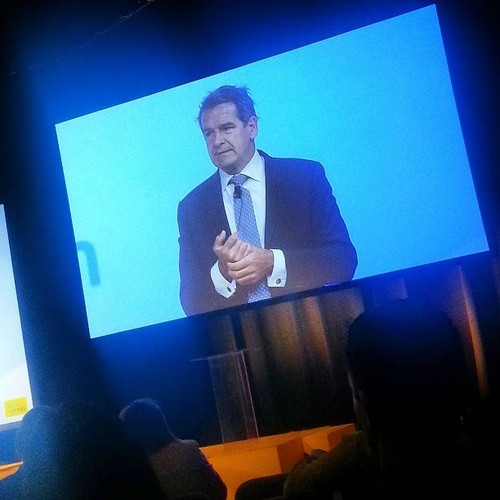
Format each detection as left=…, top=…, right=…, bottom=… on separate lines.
left=235, top=185, right=242, bottom=196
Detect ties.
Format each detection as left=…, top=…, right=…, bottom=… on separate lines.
left=227, top=174, right=271, bottom=303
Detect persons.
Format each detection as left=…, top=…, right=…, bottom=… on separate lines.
left=283, top=296, right=500, bottom=500
left=177, top=86, right=358, bottom=317
left=120, top=400, right=228, bottom=500
left=0, top=405, right=110, bottom=500
